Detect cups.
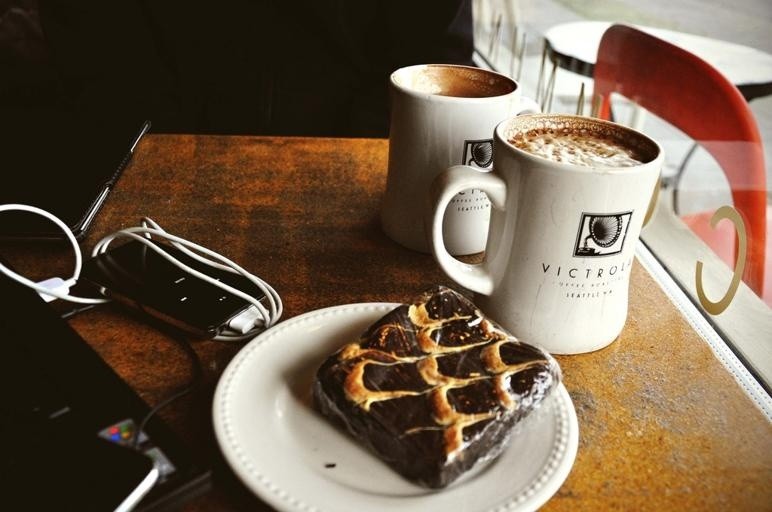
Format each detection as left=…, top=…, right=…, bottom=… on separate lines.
left=378, top=59, right=541, bottom=262
left=427, top=110, right=667, bottom=355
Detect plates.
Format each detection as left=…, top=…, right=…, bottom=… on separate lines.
left=209, top=303, right=579, bottom=512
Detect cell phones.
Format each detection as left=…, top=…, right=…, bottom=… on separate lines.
left=80, top=236, right=266, bottom=339
left=0, top=411, right=159, bottom=512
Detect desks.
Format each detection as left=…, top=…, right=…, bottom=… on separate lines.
left=533, top=18, right=772, bottom=115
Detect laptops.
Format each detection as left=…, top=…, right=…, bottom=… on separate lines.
left=0, top=254, right=212, bottom=511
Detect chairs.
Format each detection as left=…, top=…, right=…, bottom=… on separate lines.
left=591, top=23, right=768, bottom=298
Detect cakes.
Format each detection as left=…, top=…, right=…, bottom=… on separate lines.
left=313, top=284, right=562, bottom=489
